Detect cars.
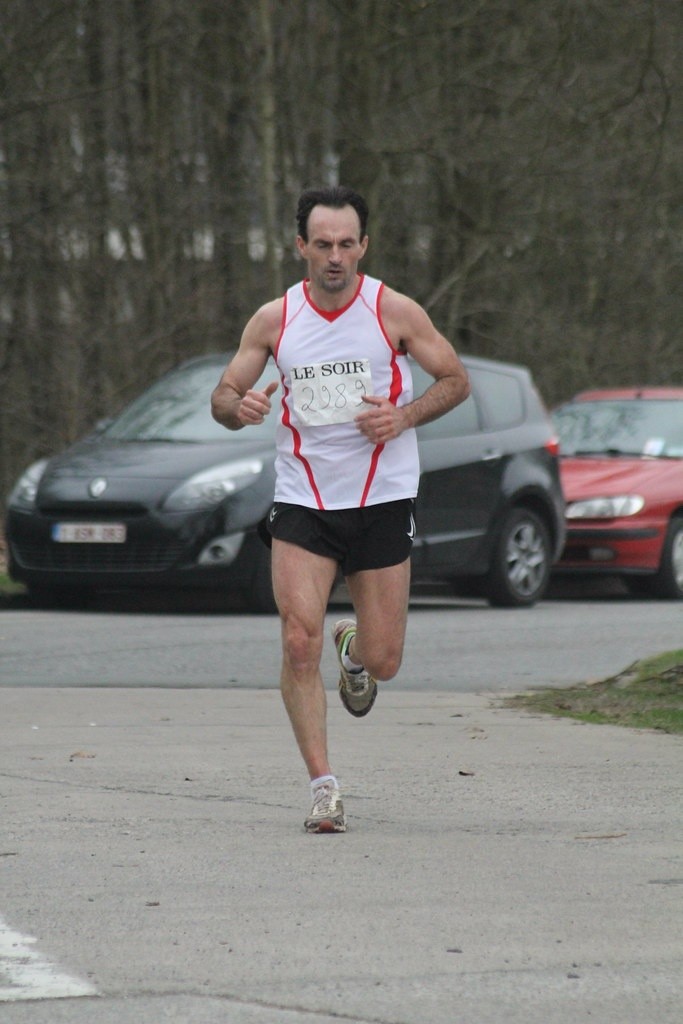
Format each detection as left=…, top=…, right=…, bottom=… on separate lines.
left=532, top=383, right=683, bottom=600
left=0, top=340, right=570, bottom=614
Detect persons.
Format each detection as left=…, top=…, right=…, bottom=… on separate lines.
left=211, top=189, right=473, bottom=832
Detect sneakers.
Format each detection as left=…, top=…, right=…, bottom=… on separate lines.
left=304, top=778, right=348, bottom=833
left=332, top=620, right=377, bottom=717
